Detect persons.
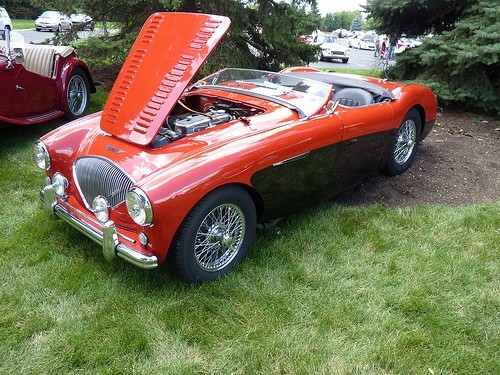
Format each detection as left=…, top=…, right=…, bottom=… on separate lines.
left=374, top=34, right=381, bottom=57
left=380, top=38, right=388, bottom=59
left=387, top=33, right=399, bottom=60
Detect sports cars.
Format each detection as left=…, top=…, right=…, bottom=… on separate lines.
left=33, top=11, right=438, bottom=280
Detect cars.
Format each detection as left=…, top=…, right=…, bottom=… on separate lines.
left=69, top=13, right=95, bottom=31
left=0, top=29, right=95, bottom=126
left=35, top=11, right=73, bottom=32
left=334, top=27, right=422, bottom=50
left=309, top=34, right=351, bottom=63
left=0, top=6, right=12, bottom=41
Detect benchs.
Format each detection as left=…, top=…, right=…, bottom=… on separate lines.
left=0, top=40, right=56, bottom=77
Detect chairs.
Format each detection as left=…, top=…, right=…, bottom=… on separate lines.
left=331, top=88, right=374, bottom=107
